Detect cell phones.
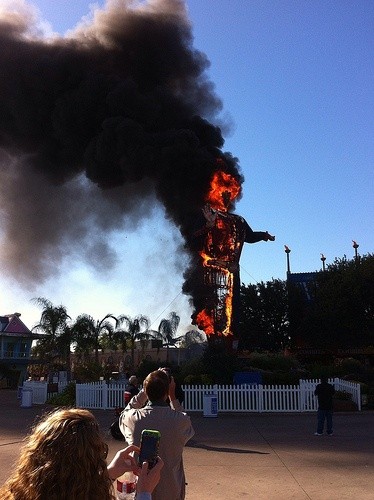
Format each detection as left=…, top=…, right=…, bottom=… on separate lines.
left=137, top=429, right=161, bottom=471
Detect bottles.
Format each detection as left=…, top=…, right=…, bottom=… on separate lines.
left=116, top=459, right=137, bottom=500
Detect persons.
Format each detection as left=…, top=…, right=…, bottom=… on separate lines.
left=0, top=408, right=163, bottom=500
left=119, top=370, right=195, bottom=500
left=313, top=377, right=335, bottom=436
left=123, top=367, right=185, bottom=409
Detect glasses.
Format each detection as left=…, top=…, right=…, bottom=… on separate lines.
left=103, top=443, right=109, bottom=459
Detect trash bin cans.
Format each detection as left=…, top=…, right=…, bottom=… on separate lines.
left=203, top=394, right=218, bottom=417
left=20, top=390, right=32, bottom=408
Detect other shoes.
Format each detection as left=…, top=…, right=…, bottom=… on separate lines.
left=314, top=432, right=323, bottom=436
left=329, top=432, right=335, bottom=437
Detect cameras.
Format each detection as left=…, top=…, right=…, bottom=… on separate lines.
left=161, top=368, right=171, bottom=384
